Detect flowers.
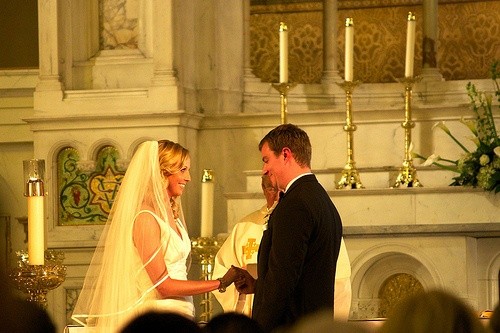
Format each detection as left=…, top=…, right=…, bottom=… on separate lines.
left=261, top=207, right=277, bottom=228
left=406, top=58, right=500, bottom=192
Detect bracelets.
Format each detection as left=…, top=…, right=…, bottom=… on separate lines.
left=216, top=278, right=226, bottom=293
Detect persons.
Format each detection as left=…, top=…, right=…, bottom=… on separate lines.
left=0, top=264, right=500, bottom=333
left=70, top=139, right=244, bottom=333
left=209, top=171, right=354, bottom=323
left=231, top=123, right=343, bottom=333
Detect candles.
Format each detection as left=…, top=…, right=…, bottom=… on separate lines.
left=345, top=18, right=354, bottom=81
left=200, top=168, right=215, bottom=239
left=405, top=12, right=417, bottom=77
left=277, top=20, right=290, bottom=84
left=23, top=157, right=46, bottom=267
